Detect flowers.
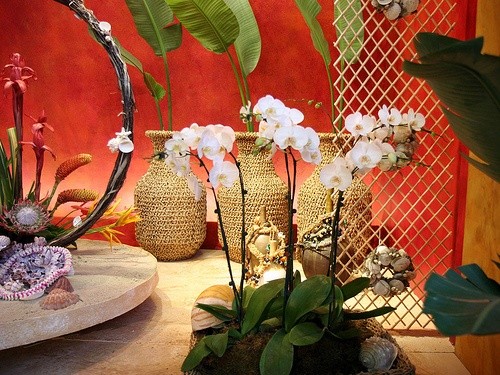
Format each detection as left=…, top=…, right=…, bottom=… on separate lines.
left=1, top=0, right=444, bottom=375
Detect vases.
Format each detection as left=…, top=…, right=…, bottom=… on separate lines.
left=218, top=133, right=290, bottom=264
left=295, top=133, right=373, bottom=266
left=134, top=130, right=207, bottom=262
